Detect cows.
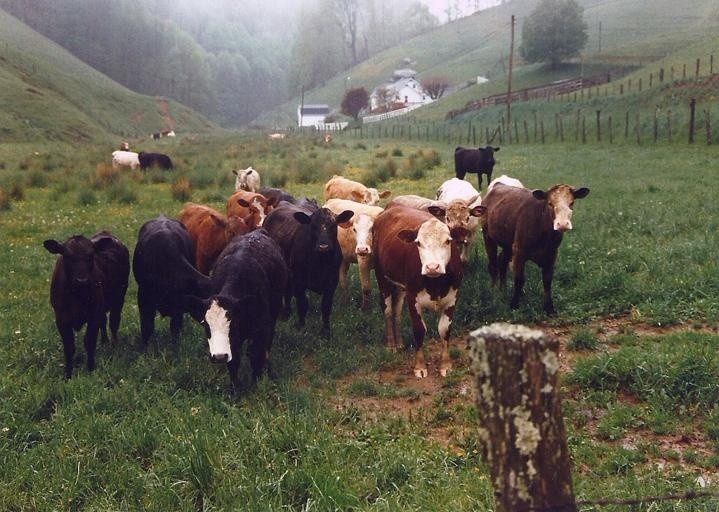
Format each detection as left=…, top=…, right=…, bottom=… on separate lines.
left=111, top=142, right=174, bottom=175
left=133, top=167, right=590, bottom=405
left=455, top=146, right=500, bottom=191
left=43, top=228, right=130, bottom=383
